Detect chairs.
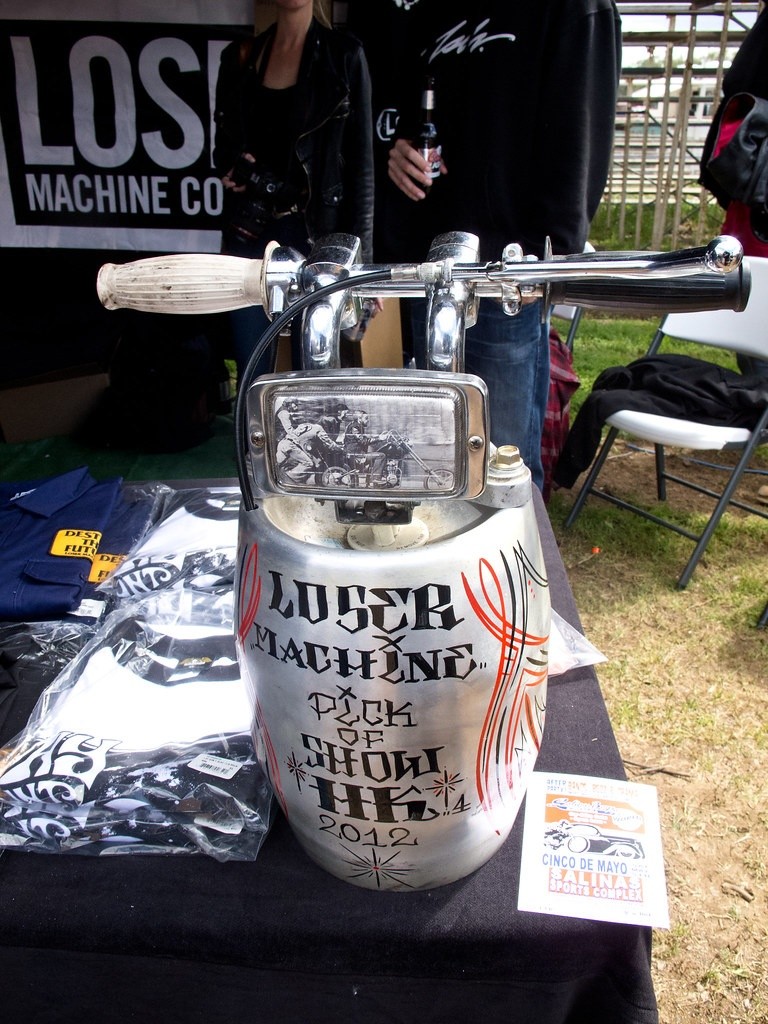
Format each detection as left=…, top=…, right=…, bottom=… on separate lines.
left=549, top=300, right=584, bottom=356
left=564, top=255, right=768, bottom=588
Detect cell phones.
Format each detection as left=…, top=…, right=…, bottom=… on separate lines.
left=230, top=156, right=283, bottom=201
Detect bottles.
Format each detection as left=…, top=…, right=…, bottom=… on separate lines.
left=409, top=76, right=445, bottom=199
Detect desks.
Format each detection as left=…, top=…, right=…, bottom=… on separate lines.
left=0, top=480, right=660, bottom=1024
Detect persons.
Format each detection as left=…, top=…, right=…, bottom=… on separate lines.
left=346, top=0, right=621, bottom=497
left=698, top=0, right=768, bottom=444
left=213, top=0, right=374, bottom=460
left=276, top=398, right=388, bottom=486
